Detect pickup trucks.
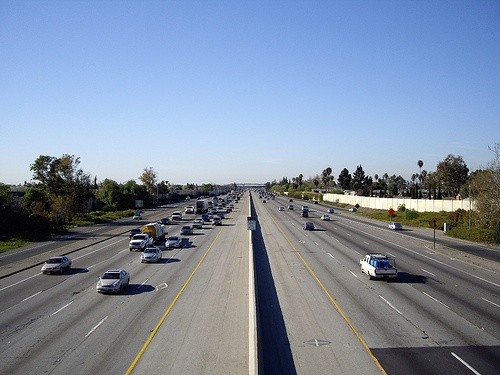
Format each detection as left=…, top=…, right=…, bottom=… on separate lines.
left=128, top=234, right=153, bottom=251
left=359, top=255, right=398, bottom=280
left=388, top=222, right=402, bottom=230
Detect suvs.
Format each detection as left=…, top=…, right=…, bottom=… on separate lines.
left=302, top=222, right=314, bottom=230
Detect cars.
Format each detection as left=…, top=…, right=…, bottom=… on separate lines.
left=141, top=246, right=162, bottom=263
left=289, top=199, right=293, bottom=202
left=321, top=215, right=330, bottom=221
left=254, top=188, right=275, bottom=203
left=300, top=206, right=309, bottom=217
left=128, top=229, right=142, bottom=241
left=96, top=269, right=130, bottom=294
left=327, top=208, right=335, bottom=213
left=157, top=189, right=244, bottom=235
left=279, top=207, right=284, bottom=211
left=41, top=257, right=71, bottom=274
left=165, top=237, right=182, bottom=248
left=132, top=214, right=142, bottom=220
left=288, top=205, right=294, bottom=210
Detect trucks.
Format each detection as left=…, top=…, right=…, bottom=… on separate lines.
left=195, top=200, right=208, bottom=214
